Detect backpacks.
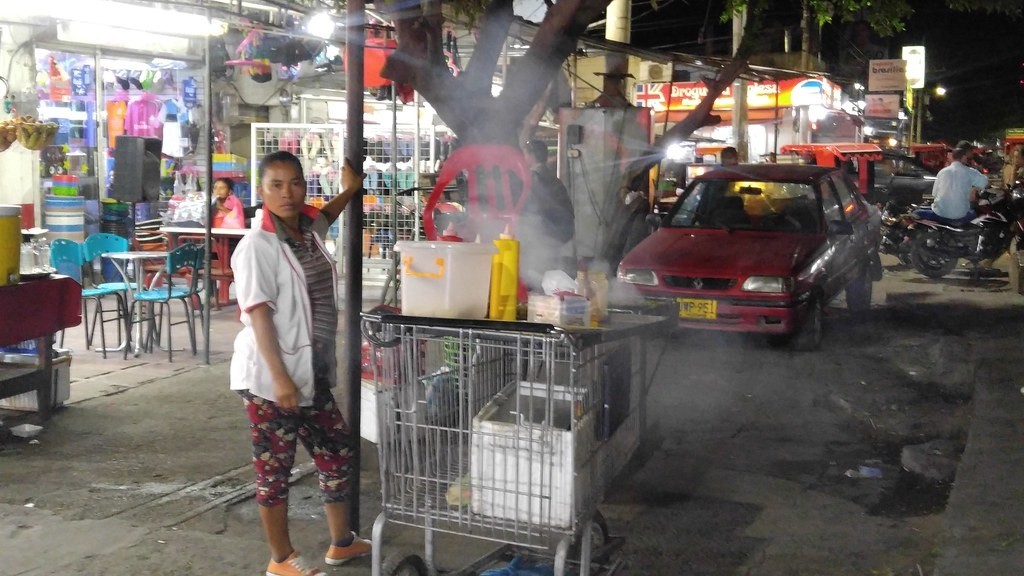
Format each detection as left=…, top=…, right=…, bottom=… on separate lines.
left=534, top=167, right=575, bottom=243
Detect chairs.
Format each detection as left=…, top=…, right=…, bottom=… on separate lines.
left=154, top=241, right=209, bottom=355
left=135, top=218, right=260, bottom=311
left=85, top=232, right=131, bottom=355
left=48, top=237, right=129, bottom=349
left=423, top=140, right=535, bottom=300
left=717, top=195, right=750, bottom=224
left=139, top=240, right=198, bottom=357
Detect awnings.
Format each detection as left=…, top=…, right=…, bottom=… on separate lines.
left=439, top=0, right=865, bottom=216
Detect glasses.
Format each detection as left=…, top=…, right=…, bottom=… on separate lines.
left=524, top=140, right=534, bottom=152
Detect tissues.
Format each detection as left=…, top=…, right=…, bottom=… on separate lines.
left=526, top=269, right=591, bottom=328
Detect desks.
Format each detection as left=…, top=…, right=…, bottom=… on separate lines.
left=157, top=223, right=259, bottom=303
left=102, top=245, right=167, bottom=357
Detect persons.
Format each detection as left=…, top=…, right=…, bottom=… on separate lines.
left=715, top=147, right=739, bottom=194
left=913, top=140, right=1024, bottom=228
left=211, top=177, right=246, bottom=304
left=229, top=151, right=372, bottom=576
left=521, top=139, right=569, bottom=258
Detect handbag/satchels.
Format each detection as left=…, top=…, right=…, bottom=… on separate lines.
left=171, top=191, right=206, bottom=228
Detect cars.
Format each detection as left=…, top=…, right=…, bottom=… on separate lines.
left=617, top=164, right=882, bottom=350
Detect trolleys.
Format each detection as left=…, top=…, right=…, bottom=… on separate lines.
left=359, top=305, right=671, bottom=575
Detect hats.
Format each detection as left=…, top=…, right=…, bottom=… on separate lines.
left=956, top=140, right=978, bottom=150
left=593, top=64, right=636, bottom=79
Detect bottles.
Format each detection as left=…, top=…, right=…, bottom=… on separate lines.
left=437, top=220, right=463, bottom=245
left=575, top=269, right=610, bottom=323
left=488, top=226, right=518, bottom=320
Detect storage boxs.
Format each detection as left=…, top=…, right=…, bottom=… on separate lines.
left=0, top=347, right=72, bottom=412
left=472, top=381, right=594, bottom=523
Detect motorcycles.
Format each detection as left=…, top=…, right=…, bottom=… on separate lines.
left=782, top=142, right=1024, bottom=280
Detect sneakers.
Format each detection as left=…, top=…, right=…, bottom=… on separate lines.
left=325, top=531, right=372, bottom=565
left=266, top=551, right=327, bottom=576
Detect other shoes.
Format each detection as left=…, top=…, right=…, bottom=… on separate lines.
left=279, top=128, right=427, bottom=258
